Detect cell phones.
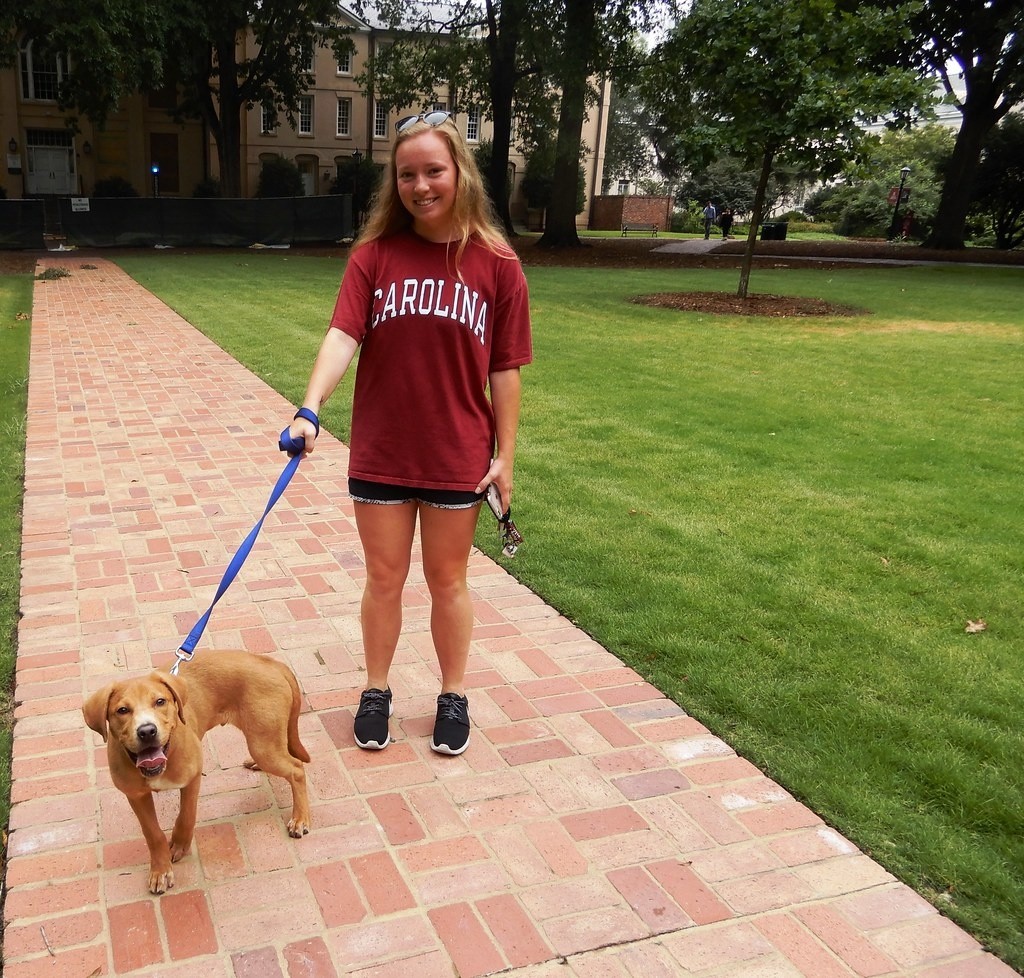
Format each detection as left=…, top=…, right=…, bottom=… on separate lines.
left=485, top=458, right=504, bottom=520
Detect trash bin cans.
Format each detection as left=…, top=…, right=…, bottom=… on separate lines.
left=760, top=221, right=789, bottom=240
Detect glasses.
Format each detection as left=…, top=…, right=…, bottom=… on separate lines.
left=395, top=109, right=454, bottom=136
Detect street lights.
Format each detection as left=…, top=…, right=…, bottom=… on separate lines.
left=886, top=166, right=910, bottom=241
left=352, top=147, right=363, bottom=240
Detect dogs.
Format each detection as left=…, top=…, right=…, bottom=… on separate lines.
left=81, top=647, right=312, bottom=895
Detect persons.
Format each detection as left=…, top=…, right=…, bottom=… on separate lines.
left=703, top=200, right=715, bottom=240
left=719, top=208, right=733, bottom=241
left=287, top=111, right=533, bottom=758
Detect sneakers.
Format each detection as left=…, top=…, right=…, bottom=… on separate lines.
left=430, top=693, right=471, bottom=754
left=353, top=684, right=393, bottom=749
